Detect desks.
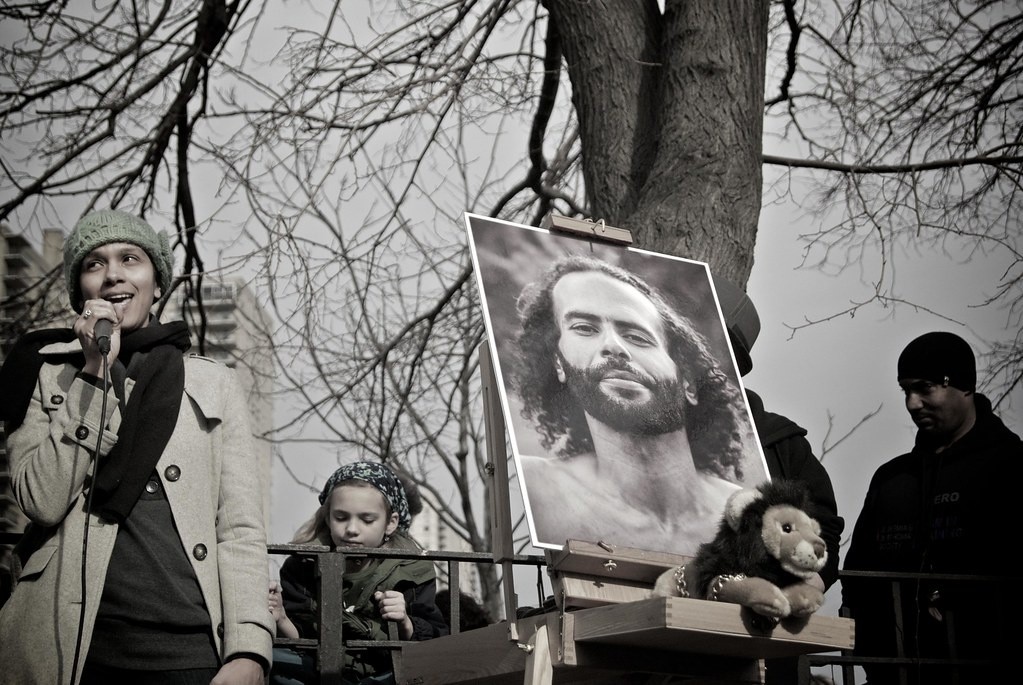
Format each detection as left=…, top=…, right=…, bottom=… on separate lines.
left=399, top=597, right=854, bottom=685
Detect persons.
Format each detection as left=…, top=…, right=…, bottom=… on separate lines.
left=840, top=327, right=1023, bottom=685
left=0, top=207, right=273, bottom=685
left=690, top=285, right=844, bottom=594
left=425, top=571, right=568, bottom=685
left=512, top=251, right=772, bottom=559
left=268, top=460, right=450, bottom=684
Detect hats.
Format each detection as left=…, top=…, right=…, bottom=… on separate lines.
left=711, top=273, right=761, bottom=377
left=63, top=209, right=174, bottom=314
left=897, top=331, right=977, bottom=390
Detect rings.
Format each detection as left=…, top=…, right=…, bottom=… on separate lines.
left=82, top=308, right=92, bottom=319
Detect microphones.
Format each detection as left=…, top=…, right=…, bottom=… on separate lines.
left=92, top=318, right=112, bottom=356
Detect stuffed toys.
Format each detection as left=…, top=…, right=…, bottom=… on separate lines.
left=649, top=485, right=832, bottom=618
